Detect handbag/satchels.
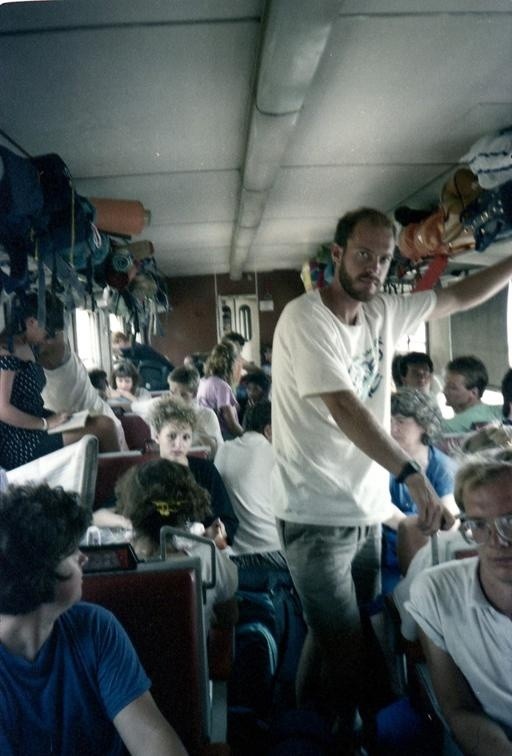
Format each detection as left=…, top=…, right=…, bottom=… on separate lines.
left=1, top=144, right=174, bottom=314
left=299, top=134, right=510, bottom=292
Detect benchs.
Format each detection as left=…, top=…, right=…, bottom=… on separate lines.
left=61, top=390, right=236, bottom=756
left=368, top=429, right=512, bottom=756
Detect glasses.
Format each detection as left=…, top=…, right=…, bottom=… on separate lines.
left=458, top=513, right=512, bottom=547
